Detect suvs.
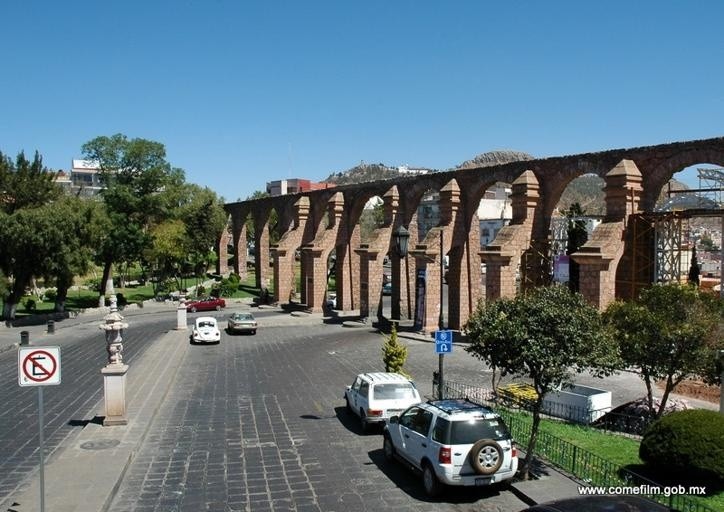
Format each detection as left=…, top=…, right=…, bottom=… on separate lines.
left=342, top=371, right=422, bottom=431
left=378, top=395, right=521, bottom=496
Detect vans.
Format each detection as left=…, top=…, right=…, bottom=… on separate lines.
left=382, top=272, right=391, bottom=283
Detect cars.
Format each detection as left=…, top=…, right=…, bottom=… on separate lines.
left=480, top=263, right=486, bottom=273
left=190, top=316, right=220, bottom=345
left=227, top=312, right=255, bottom=334
left=382, top=282, right=391, bottom=294
left=515, top=269, right=520, bottom=280
left=185, top=295, right=224, bottom=313
left=325, top=292, right=336, bottom=308
